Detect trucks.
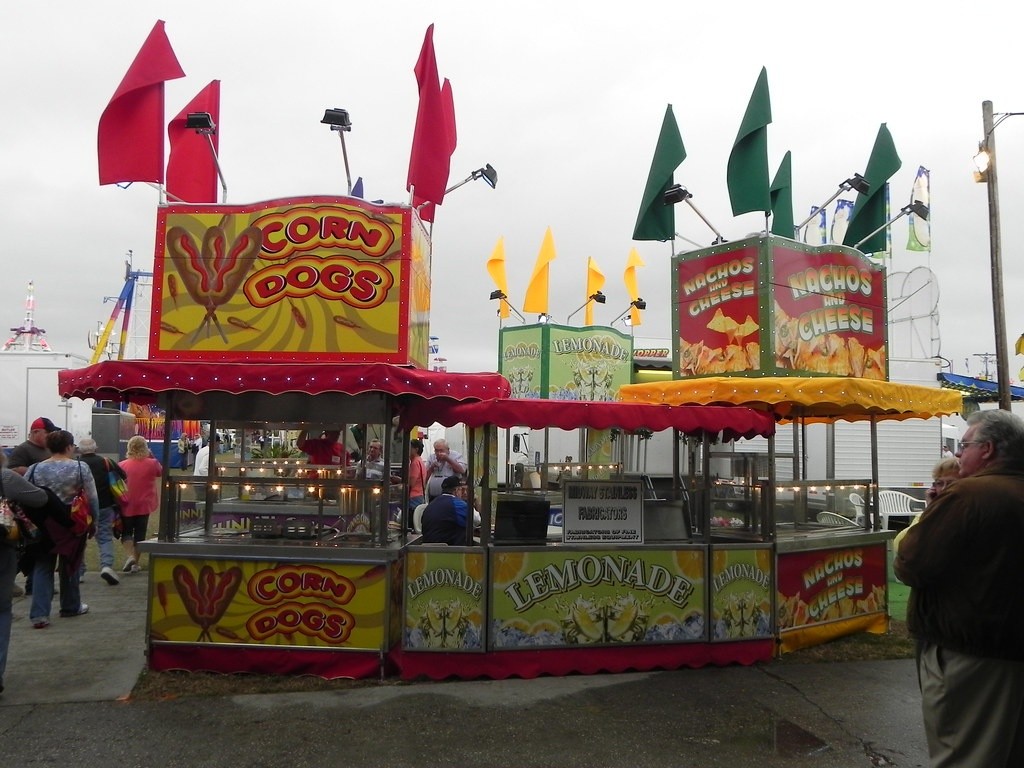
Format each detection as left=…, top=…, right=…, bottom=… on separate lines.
left=512, top=354, right=944, bottom=530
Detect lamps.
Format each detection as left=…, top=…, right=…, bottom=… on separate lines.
left=416, top=163, right=499, bottom=215
left=489, top=290, right=647, bottom=328
left=661, top=233, right=704, bottom=247
left=793, top=173, right=871, bottom=242
left=113, top=182, right=183, bottom=205
left=186, top=111, right=229, bottom=205
left=320, top=109, right=354, bottom=197
left=856, top=200, right=932, bottom=254
left=666, top=184, right=728, bottom=248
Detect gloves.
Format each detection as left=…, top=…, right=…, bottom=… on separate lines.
left=87, top=525, right=96, bottom=540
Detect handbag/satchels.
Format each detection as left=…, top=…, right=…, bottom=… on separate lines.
left=63, top=488, right=93, bottom=536
left=113, top=512, right=123, bottom=539
left=104, top=456, right=128, bottom=507
left=0, top=498, right=39, bottom=548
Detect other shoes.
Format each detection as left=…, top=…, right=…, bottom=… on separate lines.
left=13, top=585, right=24, bottom=597
left=100, top=567, right=119, bottom=585
left=60, top=602, right=89, bottom=616
left=29, top=617, right=48, bottom=629
left=24, top=585, right=58, bottom=594
left=122, top=555, right=136, bottom=572
left=131, top=565, right=142, bottom=573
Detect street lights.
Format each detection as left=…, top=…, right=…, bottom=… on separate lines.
left=973, top=146, right=1013, bottom=410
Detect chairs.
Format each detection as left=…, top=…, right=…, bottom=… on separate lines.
left=816, top=491, right=928, bottom=531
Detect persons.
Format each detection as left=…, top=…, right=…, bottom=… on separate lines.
left=297, top=418, right=353, bottom=500
left=118, top=435, right=163, bottom=573
left=74, top=441, right=122, bottom=587
left=0, top=409, right=488, bottom=697
left=21, top=431, right=98, bottom=626
left=10, top=417, right=61, bottom=474
left=0, top=446, right=50, bottom=688
left=178, top=432, right=188, bottom=472
left=420, top=476, right=483, bottom=549
left=895, top=407, right=1024, bottom=768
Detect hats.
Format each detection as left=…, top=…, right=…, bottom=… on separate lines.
left=207, top=434, right=221, bottom=442
left=30, top=417, right=61, bottom=432
left=441, top=476, right=466, bottom=488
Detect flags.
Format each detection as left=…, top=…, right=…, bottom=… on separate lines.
left=97, top=19, right=186, bottom=187
left=486, top=235, right=510, bottom=319
left=624, top=249, right=643, bottom=325
left=409, top=80, right=459, bottom=223
left=770, top=149, right=795, bottom=239
left=632, top=103, right=689, bottom=241
left=584, top=258, right=606, bottom=324
left=844, top=124, right=900, bottom=257
left=522, top=225, right=556, bottom=314
left=166, top=80, right=220, bottom=202
left=725, top=66, right=773, bottom=217
left=406, top=19, right=444, bottom=201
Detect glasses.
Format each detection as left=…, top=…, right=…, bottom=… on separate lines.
left=956, top=439, right=984, bottom=452
